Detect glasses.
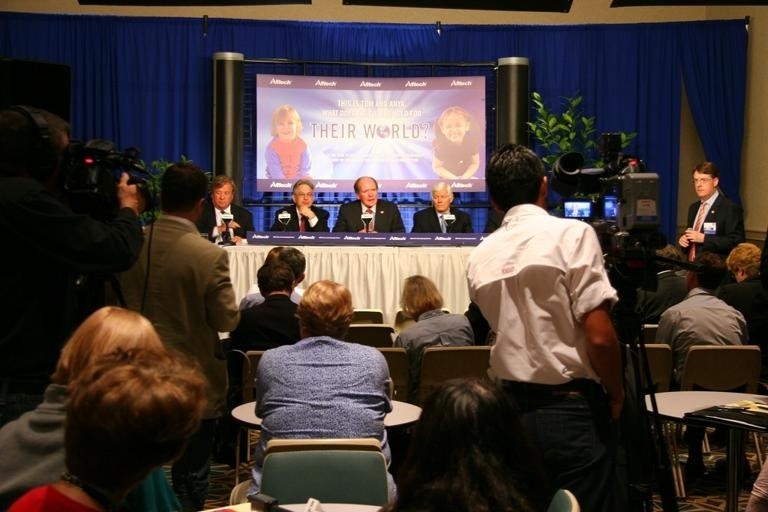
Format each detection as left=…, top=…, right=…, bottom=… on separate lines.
left=694, top=178, right=714, bottom=184
left=298, top=191, right=313, bottom=198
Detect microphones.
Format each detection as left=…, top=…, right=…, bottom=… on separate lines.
left=221, top=211, right=232, bottom=223
left=443, top=213, right=456, bottom=228
left=360, top=212, right=373, bottom=227
left=278, top=211, right=290, bottom=228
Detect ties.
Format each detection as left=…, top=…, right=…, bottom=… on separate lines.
left=366, top=208, right=374, bottom=232
left=688, top=202, right=708, bottom=262
left=220, top=210, right=232, bottom=242
left=300, top=214, right=308, bottom=232
left=440, top=215, right=447, bottom=233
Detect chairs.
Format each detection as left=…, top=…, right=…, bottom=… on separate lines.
left=644, top=322, right=660, bottom=345
left=394, top=308, right=451, bottom=338
left=419, top=345, right=496, bottom=408
left=376, top=347, right=411, bottom=404
left=242, top=349, right=266, bottom=465
left=260, top=452, right=391, bottom=512
left=352, top=310, right=383, bottom=324
left=347, top=323, right=394, bottom=347
left=626, top=343, right=680, bottom=499
left=264, top=437, right=383, bottom=455
left=679, top=343, right=764, bottom=501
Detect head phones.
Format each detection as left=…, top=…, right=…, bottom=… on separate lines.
left=7, top=104, right=68, bottom=192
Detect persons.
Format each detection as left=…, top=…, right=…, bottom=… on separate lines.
left=221, top=265, right=301, bottom=352
left=632, top=253, right=687, bottom=325
left=677, top=162, right=744, bottom=261
left=105, top=161, right=241, bottom=512
left=0, top=307, right=185, bottom=512
left=229, top=281, right=398, bottom=512
left=0, top=104, right=143, bottom=423
left=744, top=451, right=768, bottom=512
left=8, top=347, right=211, bottom=512
left=467, top=142, right=625, bottom=512
left=410, top=182, right=473, bottom=234
left=238, top=248, right=305, bottom=308
left=333, top=177, right=404, bottom=233
left=196, top=176, right=254, bottom=246
left=271, top=180, right=330, bottom=233
left=265, top=107, right=312, bottom=183
left=395, top=276, right=474, bottom=382
left=655, top=253, right=748, bottom=498
left=718, top=243, right=768, bottom=354
left=432, top=106, right=480, bottom=179
left=398, top=378, right=541, bottom=511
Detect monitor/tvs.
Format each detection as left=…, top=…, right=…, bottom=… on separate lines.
left=561, top=199, right=593, bottom=220
left=602, top=196, right=619, bottom=221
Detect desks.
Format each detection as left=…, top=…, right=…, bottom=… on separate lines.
left=645, top=389, right=768, bottom=512
left=194, top=500, right=388, bottom=511
left=232, top=397, right=422, bottom=426
left=215, top=245, right=477, bottom=341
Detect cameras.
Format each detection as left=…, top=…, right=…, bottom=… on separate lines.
left=63, top=140, right=155, bottom=220
left=551, top=132, right=663, bottom=232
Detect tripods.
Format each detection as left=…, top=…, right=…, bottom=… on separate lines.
left=601, top=242, right=704, bottom=511
